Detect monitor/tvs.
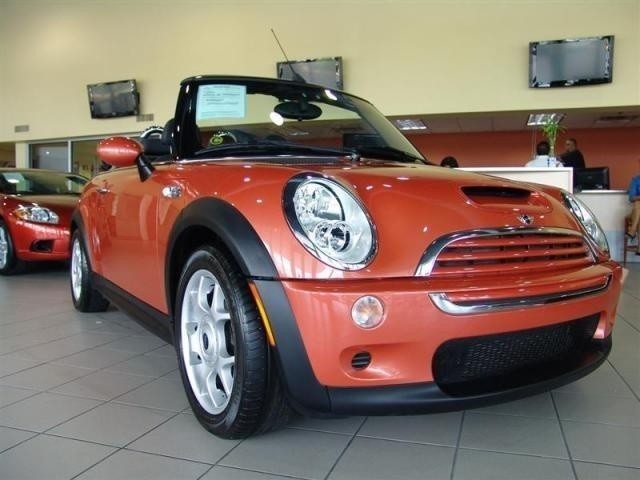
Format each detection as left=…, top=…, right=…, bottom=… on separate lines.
left=574, top=166, right=610, bottom=190
left=528, top=35, right=614, bottom=88
left=276, top=57, right=343, bottom=103
left=87, top=79, right=139, bottom=118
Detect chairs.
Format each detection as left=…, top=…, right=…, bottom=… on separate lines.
left=162, top=118, right=205, bottom=154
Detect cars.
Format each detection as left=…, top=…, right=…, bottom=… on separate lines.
left=0, top=165, right=92, bottom=277
left=65, top=26, right=627, bottom=438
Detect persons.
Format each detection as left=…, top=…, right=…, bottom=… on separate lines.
left=559, top=138, right=585, bottom=168
left=441, top=156, right=458, bottom=167
left=524, top=141, right=563, bottom=167
left=625, top=175, right=640, bottom=256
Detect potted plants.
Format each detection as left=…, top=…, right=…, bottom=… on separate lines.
left=538, top=114, right=567, bottom=169
